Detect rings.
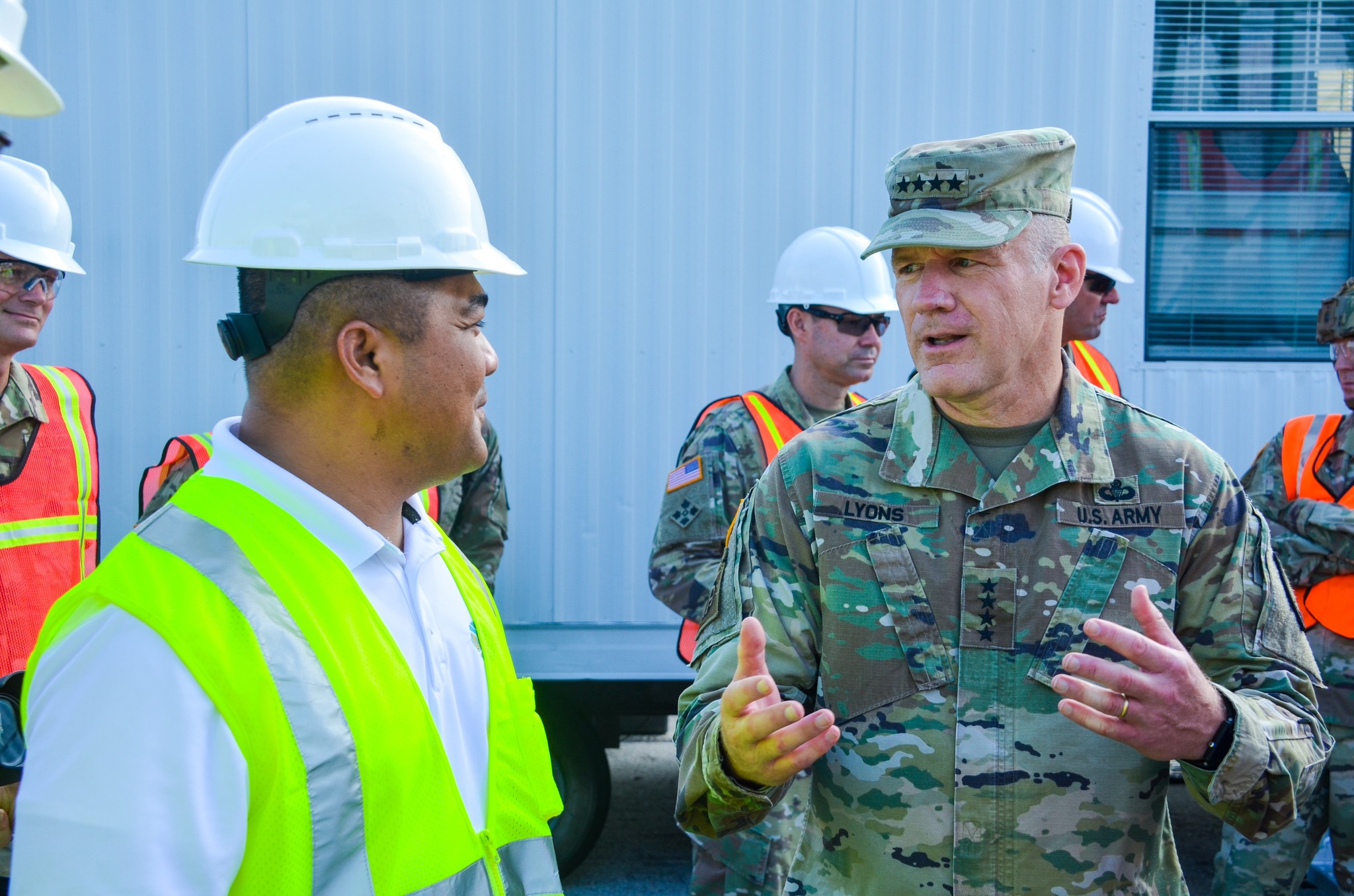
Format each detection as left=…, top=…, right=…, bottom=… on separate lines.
left=1116, top=693, right=1129, bottom=719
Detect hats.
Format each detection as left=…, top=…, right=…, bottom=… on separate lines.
left=860, top=127, right=1076, bottom=261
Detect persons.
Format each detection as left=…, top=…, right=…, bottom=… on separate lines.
left=648, top=243, right=891, bottom=624
left=1067, top=186, right=1126, bottom=399
left=0, top=169, right=101, bottom=896
left=130, top=427, right=217, bottom=524
left=404, top=413, right=510, bottom=598
left=6, top=161, right=563, bottom=895
left=671, top=156, right=1332, bottom=894
left=1209, top=276, right=1354, bottom=896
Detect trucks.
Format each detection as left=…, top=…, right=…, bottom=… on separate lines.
left=0, top=0, right=1354, bottom=883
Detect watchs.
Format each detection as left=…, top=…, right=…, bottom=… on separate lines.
left=1179, top=689, right=1235, bottom=772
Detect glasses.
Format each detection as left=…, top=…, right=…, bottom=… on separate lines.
left=1084, top=273, right=1115, bottom=294
left=807, top=307, right=890, bottom=337
left=1329, top=341, right=1354, bottom=361
left=1, top=259, right=65, bottom=299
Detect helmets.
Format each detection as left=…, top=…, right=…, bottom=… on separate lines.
left=766, top=226, right=900, bottom=314
left=0, top=153, right=87, bottom=274
left=185, top=97, right=528, bottom=276
left=1067, top=187, right=1134, bottom=283
left=0, top=0, right=64, bottom=117
left=1315, top=276, right=1354, bottom=345
